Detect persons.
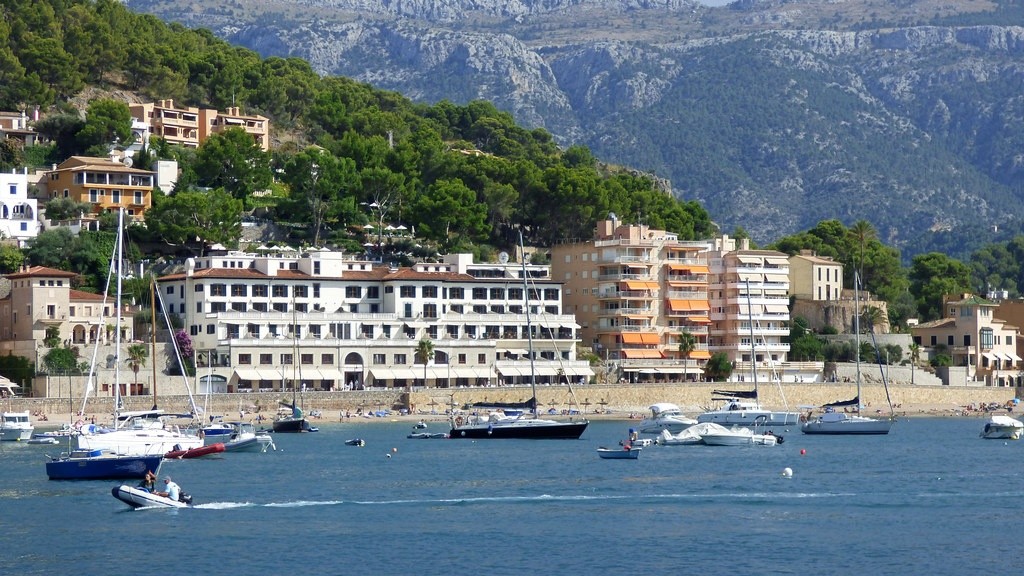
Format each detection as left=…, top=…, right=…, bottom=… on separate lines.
left=340, top=410, right=344, bottom=423
left=349, top=379, right=358, bottom=390
left=346, top=409, right=351, bottom=423
left=139, top=473, right=156, bottom=494
left=158, top=476, right=181, bottom=502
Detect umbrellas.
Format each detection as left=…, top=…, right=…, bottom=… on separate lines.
left=392, top=400, right=405, bottom=409
left=580, top=401, right=592, bottom=413
left=374, top=400, right=386, bottom=410
left=426, top=401, right=439, bottom=413
left=446, top=400, right=458, bottom=410
left=596, top=401, right=608, bottom=410
left=547, top=401, right=559, bottom=409
left=564, top=401, right=576, bottom=410
left=358, top=402, right=370, bottom=415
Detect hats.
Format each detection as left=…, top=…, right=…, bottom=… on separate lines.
left=162, top=476, right=171, bottom=481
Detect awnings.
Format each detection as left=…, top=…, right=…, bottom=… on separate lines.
left=623, top=368, right=705, bottom=374
left=982, top=353, right=1022, bottom=361
left=369, top=367, right=498, bottom=380
left=234, top=370, right=342, bottom=381
left=497, top=366, right=595, bottom=376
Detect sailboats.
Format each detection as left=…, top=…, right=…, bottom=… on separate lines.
left=272, top=299, right=310, bottom=433
left=450, top=230, right=589, bottom=439
left=199, top=349, right=235, bottom=445
left=69, top=207, right=225, bottom=459
left=795, top=271, right=898, bottom=435
left=696, top=278, right=800, bottom=426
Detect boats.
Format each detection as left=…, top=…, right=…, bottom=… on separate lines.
left=653, top=429, right=703, bottom=445
left=34, top=430, right=80, bottom=444
left=639, top=403, right=698, bottom=435
left=44, top=443, right=165, bottom=481
left=619, top=428, right=652, bottom=446
left=310, top=426, right=319, bottom=432
left=111, top=484, right=193, bottom=507
left=223, top=421, right=273, bottom=452
left=407, top=432, right=432, bottom=439
left=412, top=422, right=427, bottom=428
left=979, top=416, right=1024, bottom=439
left=0, top=412, right=34, bottom=440
left=345, top=438, right=364, bottom=446
left=699, top=415, right=785, bottom=446
left=597, top=445, right=642, bottom=459
left=431, top=433, right=449, bottom=438
left=27, top=434, right=59, bottom=445
